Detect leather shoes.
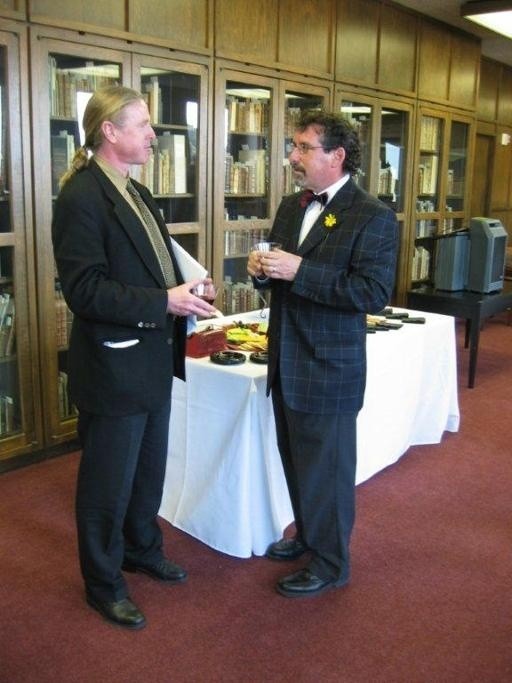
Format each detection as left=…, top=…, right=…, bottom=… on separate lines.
left=87, top=596, right=147, bottom=626
left=121, top=554, right=187, bottom=584
left=266, top=537, right=310, bottom=561
left=276, top=567, right=349, bottom=596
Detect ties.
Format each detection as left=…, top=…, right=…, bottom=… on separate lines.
left=126, top=181, right=178, bottom=289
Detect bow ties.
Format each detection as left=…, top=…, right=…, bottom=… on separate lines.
left=298, top=188, right=329, bottom=208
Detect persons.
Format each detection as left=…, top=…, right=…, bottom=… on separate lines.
left=51, top=87, right=221, bottom=631
left=245, top=109, right=399, bottom=598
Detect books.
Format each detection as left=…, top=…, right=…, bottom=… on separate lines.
left=54, top=286, right=82, bottom=345
left=283, top=159, right=312, bottom=195
left=56, top=368, right=89, bottom=421
left=220, top=276, right=278, bottom=315
left=440, top=217, right=462, bottom=246
left=49, top=135, right=198, bottom=191
left=221, top=222, right=276, bottom=260
left=140, top=73, right=163, bottom=128
left=282, top=95, right=334, bottom=141
left=410, top=245, right=442, bottom=283
left=217, top=279, right=279, bottom=319
left=227, top=84, right=273, bottom=137
left=224, top=146, right=273, bottom=198
left=1, top=381, right=25, bottom=442
left=374, top=161, right=404, bottom=196
left=47, top=61, right=128, bottom=119
left=412, top=197, right=437, bottom=237
left=445, top=167, right=463, bottom=197
left=412, top=111, right=443, bottom=154
left=416, top=159, right=440, bottom=197
left=2, top=274, right=20, bottom=363
left=340, top=100, right=371, bottom=135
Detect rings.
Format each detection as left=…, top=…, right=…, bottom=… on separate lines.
left=273, top=268, right=276, bottom=272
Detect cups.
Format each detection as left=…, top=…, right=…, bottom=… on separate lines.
left=192, top=281, right=219, bottom=307
left=253, top=242, right=282, bottom=259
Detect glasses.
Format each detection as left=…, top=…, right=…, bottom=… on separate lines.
left=290, top=142, right=332, bottom=153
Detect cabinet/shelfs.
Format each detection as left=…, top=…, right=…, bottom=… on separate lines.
left=27, top=0, right=217, bottom=456
left=1, top=0, right=44, bottom=470
left=331, top=1, right=421, bottom=322
left=405, top=8, right=482, bottom=320
left=212, top=1, right=336, bottom=323
left=474, top=54, right=512, bottom=263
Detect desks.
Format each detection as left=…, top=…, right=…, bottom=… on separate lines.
left=408, top=283, right=509, bottom=390
